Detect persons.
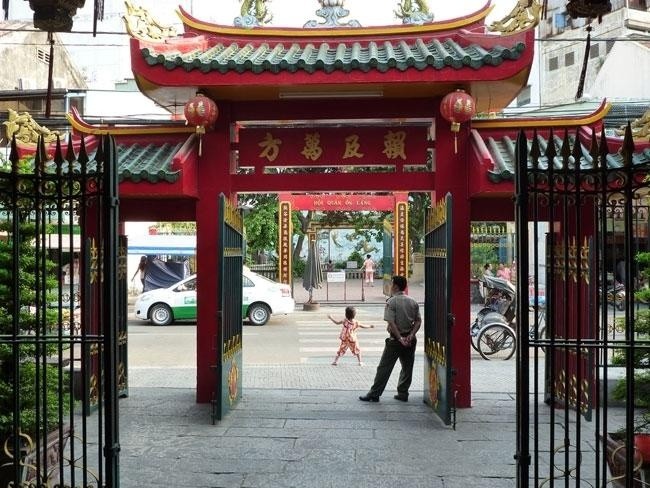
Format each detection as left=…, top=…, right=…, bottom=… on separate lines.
left=326, top=260, right=332, bottom=272
left=358, top=276, right=422, bottom=403
left=496, top=262, right=511, bottom=282
left=131, top=256, right=146, bottom=293
left=359, top=255, right=375, bottom=287
left=327, top=306, right=374, bottom=366
left=510, top=261, right=518, bottom=285
left=617, top=258, right=626, bottom=281
left=484, top=263, right=494, bottom=276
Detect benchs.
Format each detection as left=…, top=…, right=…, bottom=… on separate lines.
left=29, top=356, right=71, bottom=368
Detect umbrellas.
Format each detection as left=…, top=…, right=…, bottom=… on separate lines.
left=301, top=240, right=323, bottom=304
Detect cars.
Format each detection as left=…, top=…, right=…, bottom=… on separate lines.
left=132, top=268, right=297, bottom=327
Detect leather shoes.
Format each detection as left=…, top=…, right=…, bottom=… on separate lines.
left=359, top=396, right=379, bottom=402
left=394, top=395, right=408, bottom=401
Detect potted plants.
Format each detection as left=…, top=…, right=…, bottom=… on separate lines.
left=600, top=172, right=650, bottom=488
left=0, top=147, right=81, bottom=488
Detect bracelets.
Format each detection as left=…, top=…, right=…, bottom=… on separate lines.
left=398, top=337, right=403, bottom=341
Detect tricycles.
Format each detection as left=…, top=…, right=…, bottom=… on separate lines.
left=470, top=274, right=546, bottom=362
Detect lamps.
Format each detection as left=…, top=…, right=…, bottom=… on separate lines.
left=278, top=89, right=384, bottom=100
